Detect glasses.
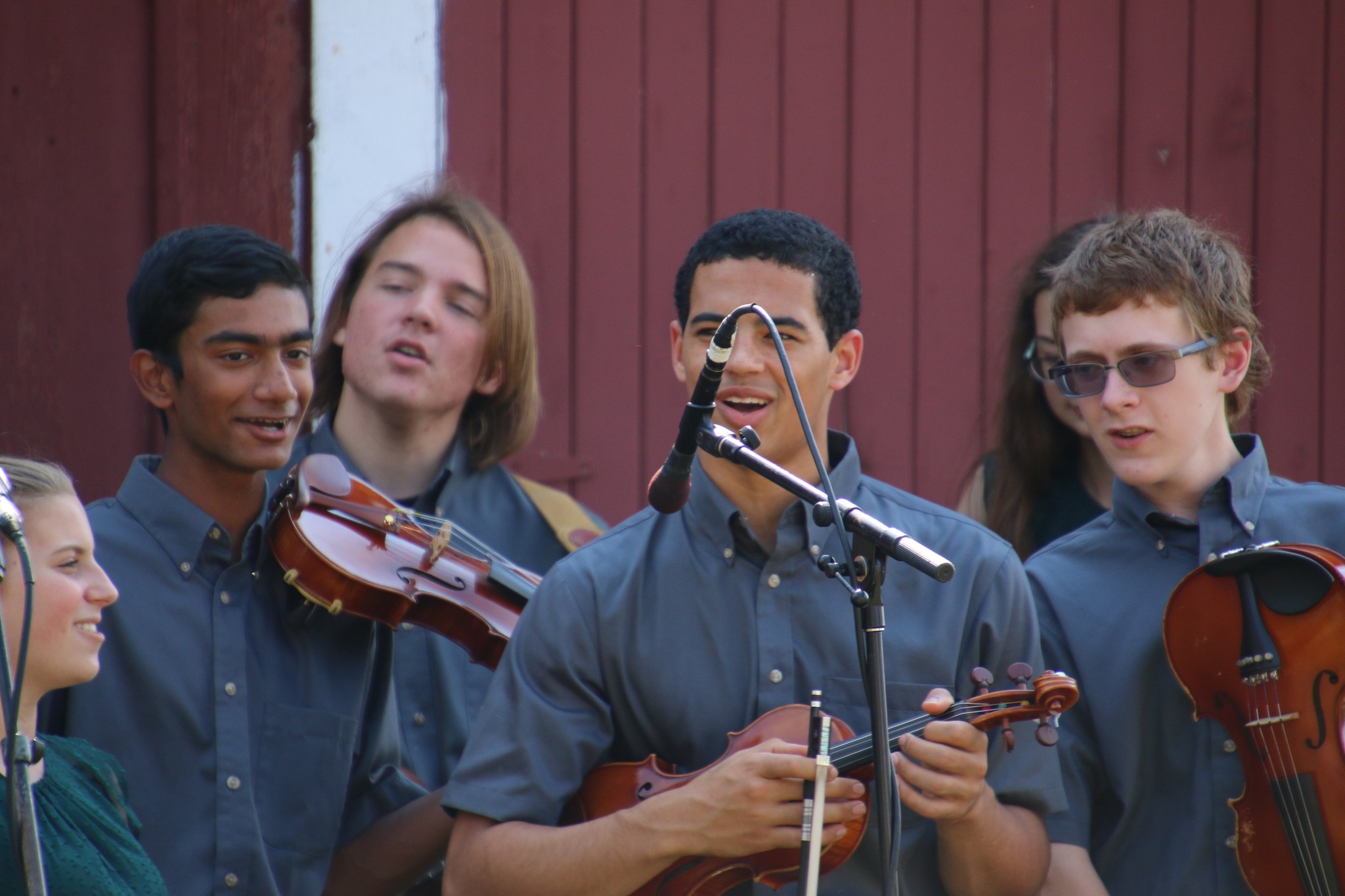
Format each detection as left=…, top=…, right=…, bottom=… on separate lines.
left=1022, top=339, right=1061, bottom=385
left=1048, top=337, right=1218, bottom=398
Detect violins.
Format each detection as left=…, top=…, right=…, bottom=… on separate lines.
left=266, top=453, right=546, bottom=674
left=560, top=662, right=1080, bottom=896
left=1163, top=540, right=1345, bottom=896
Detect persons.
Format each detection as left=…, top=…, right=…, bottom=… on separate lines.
left=265, top=177, right=611, bottom=896
left=34, top=223, right=455, bottom=896
left=1022, top=208, right=1345, bottom=896
left=0, top=455, right=169, bottom=896
left=440, top=208, right=1069, bottom=896
left=952, top=216, right=1272, bottom=565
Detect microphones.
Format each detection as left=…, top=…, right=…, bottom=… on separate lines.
left=0, top=466, right=25, bottom=542
left=646, top=313, right=739, bottom=516
